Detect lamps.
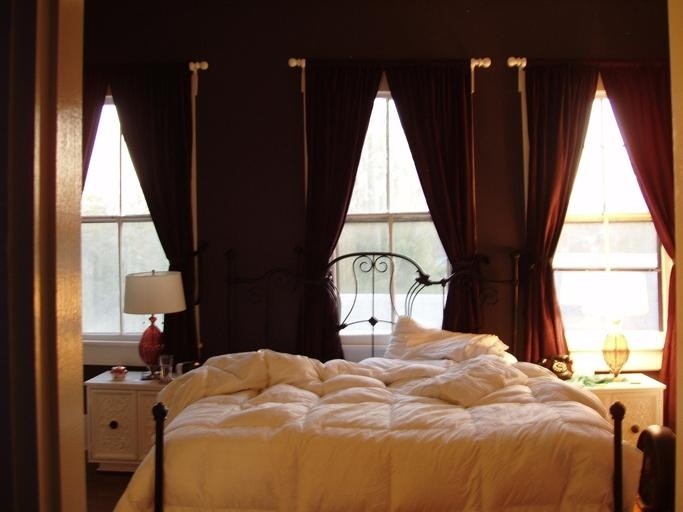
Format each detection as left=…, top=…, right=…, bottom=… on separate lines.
left=123, top=269, right=189, bottom=383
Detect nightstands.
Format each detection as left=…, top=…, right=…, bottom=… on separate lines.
left=580, top=371, right=666, bottom=438
left=83, top=367, right=178, bottom=473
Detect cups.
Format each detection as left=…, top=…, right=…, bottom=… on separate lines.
left=159, top=355, right=173, bottom=382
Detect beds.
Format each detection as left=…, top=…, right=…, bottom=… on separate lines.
left=113, top=251, right=647, bottom=508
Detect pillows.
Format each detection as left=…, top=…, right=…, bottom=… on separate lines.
left=384, top=314, right=508, bottom=365
